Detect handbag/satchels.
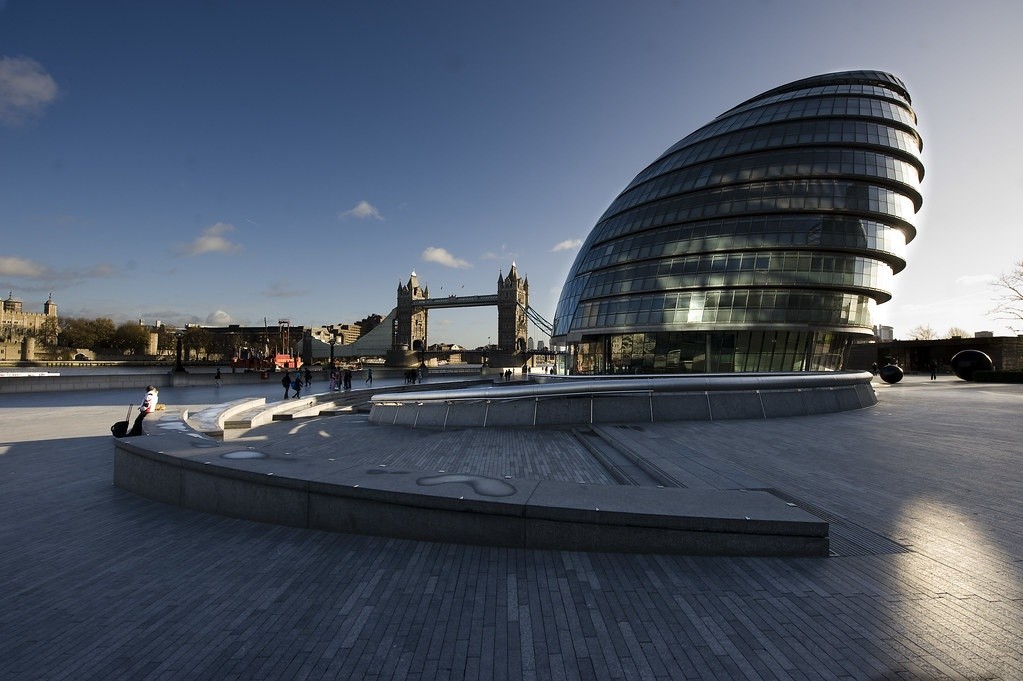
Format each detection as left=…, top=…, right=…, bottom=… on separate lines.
left=290, top=382, right=296, bottom=390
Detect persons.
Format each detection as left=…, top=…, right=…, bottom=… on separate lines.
left=872, top=361, right=878, bottom=376
left=214, top=368, right=221, bottom=388
left=304, top=367, right=312, bottom=387
left=298, top=368, right=303, bottom=379
left=404, top=369, right=422, bottom=385
left=528, top=365, right=557, bottom=379
left=504, top=369, right=512, bottom=381
left=330, top=369, right=353, bottom=391
left=124, top=385, right=159, bottom=437
left=292, top=373, right=303, bottom=399
left=365, top=367, right=373, bottom=384
left=930, top=362, right=937, bottom=381
left=282, top=372, right=291, bottom=399
